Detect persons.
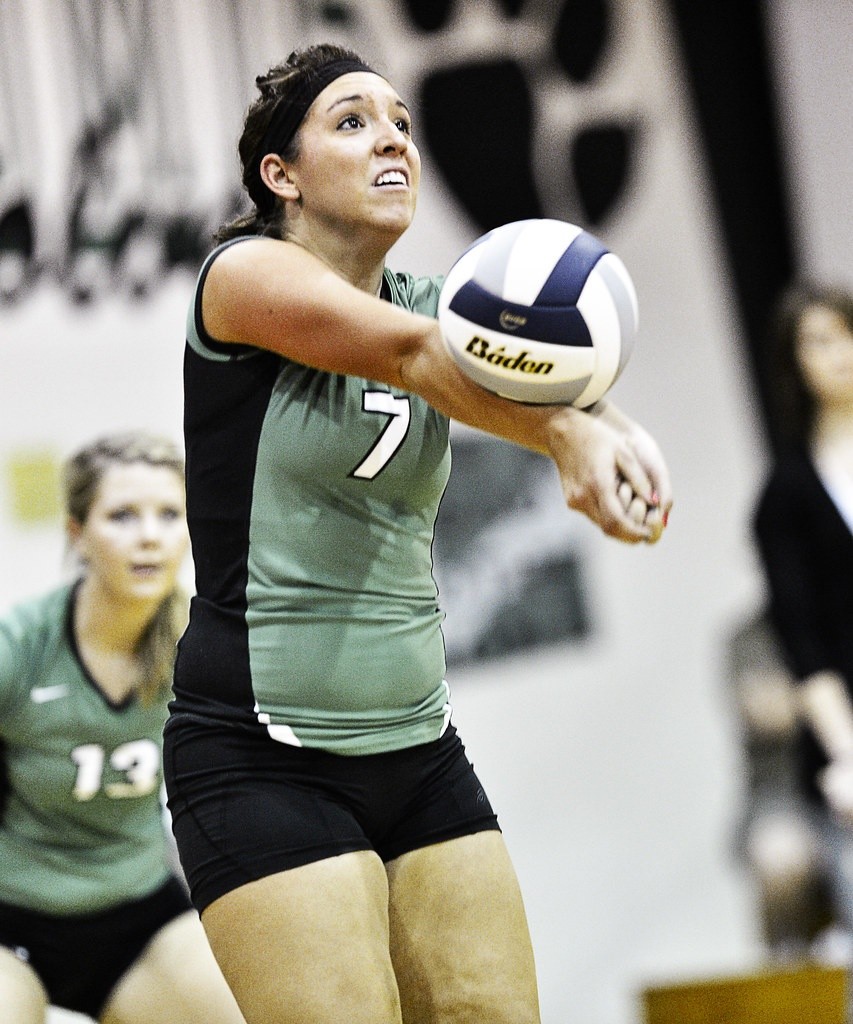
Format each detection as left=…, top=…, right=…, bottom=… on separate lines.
left=0, top=429, right=248, bottom=1024
left=161, top=40, right=672, bottom=1024
left=749, top=289, right=853, bottom=933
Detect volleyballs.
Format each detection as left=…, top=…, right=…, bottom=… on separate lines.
left=435, top=214, right=640, bottom=413
